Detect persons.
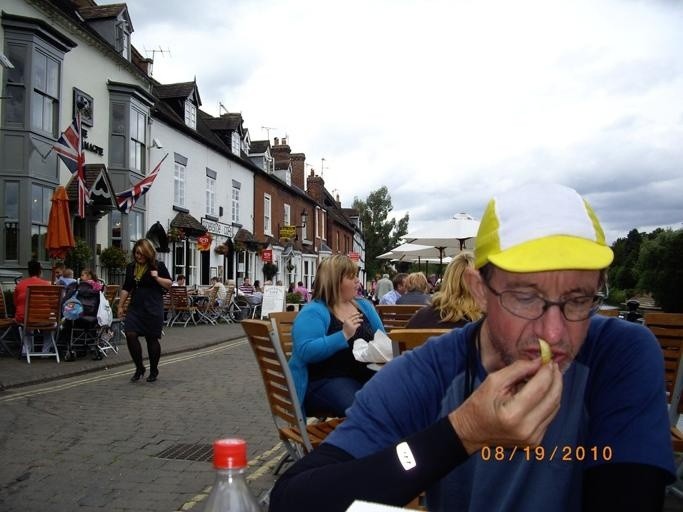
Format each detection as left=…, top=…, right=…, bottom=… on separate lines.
left=172, top=275, right=185, bottom=287
left=117, top=240, right=173, bottom=382
left=202, top=277, right=227, bottom=308
left=13, top=259, right=112, bottom=362
left=239, top=179, right=676, bottom=512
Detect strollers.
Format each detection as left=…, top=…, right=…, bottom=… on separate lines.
left=60, top=279, right=104, bottom=362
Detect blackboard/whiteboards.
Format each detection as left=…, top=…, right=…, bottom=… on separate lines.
left=262, top=285, right=285, bottom=317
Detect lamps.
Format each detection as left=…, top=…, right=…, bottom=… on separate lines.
left=290, top=206, right=309, bottom=230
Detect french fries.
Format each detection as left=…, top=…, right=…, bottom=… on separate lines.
left=540, top=338, right=551, bottom=365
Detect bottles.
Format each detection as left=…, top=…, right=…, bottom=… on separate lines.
left=199, top=438, right=260, bottom=512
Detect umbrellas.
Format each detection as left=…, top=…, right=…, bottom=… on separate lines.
left=44, top=185, right=75, bottom=286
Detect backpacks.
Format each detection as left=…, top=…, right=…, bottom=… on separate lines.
left=62, top=290, right=82, bottom=320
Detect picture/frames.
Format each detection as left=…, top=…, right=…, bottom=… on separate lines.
left=70, top=86, right=93, bottom=126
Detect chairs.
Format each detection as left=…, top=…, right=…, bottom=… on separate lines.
left=0, top=273, right=262, bottom=368
left=241, top=298, right=683, bottom=480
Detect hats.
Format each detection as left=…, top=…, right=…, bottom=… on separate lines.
left=473, top=179, right=615, bottom=274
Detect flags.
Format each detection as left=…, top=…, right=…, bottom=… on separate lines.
left=113, top=162, right=161, bottom=215
left=53, top=111, right=85, bottom=219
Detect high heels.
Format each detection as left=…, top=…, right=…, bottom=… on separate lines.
left=130, top=366, right=146, bottom=382
left=146, top=368, right=159, bottom=382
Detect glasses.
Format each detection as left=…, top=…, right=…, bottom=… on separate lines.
left=481, top=273, right=609, bottom=321
